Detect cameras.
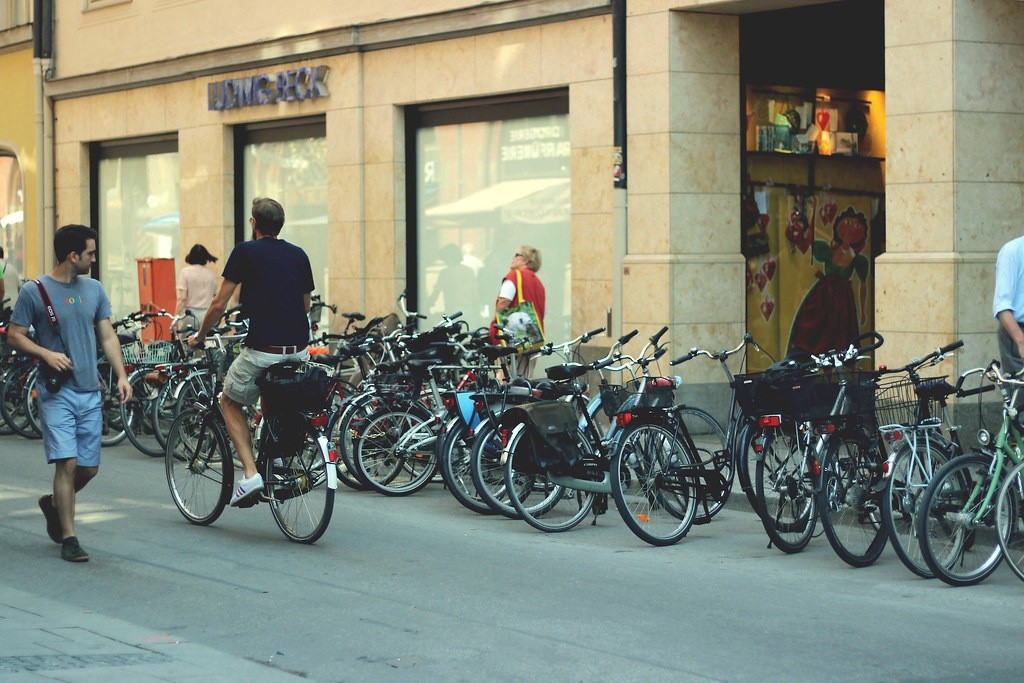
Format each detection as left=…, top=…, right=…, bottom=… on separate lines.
left=39, top=362, right=72, bottom=393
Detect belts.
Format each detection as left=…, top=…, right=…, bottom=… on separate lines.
left=247, top=344, right=308, bottom=355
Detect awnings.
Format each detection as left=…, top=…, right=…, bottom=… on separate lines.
left=424, top=175, right=570, bottom=223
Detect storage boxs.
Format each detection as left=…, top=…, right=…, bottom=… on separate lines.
left=768, top=98, right=859, bottom=155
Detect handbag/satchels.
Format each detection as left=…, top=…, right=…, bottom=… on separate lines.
left=496, top=269, right=545, bottom=354
left=503, top=399, right=585, bottom=476
left=407, top=325, right=453, bottom=365
left=749, top=358, right=798, bottom=408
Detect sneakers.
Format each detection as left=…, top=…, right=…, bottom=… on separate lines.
left=231, top=472, right=264, bottom=506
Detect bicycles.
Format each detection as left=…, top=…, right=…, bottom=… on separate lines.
left=0, top=293, right=1024, bottom=590
left=163, top=331, right=340, bottom=545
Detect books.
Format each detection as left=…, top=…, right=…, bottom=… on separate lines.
left=755, top=123, right=791, bottom=152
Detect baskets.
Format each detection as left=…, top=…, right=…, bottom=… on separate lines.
left=309, top=303, right=320, bottom=322
left=805, top=371, right=877, bottom=431
left=119, top=339, right=173, bottom=364
left=864, top=374, right=949, bottom=431
left=598, top=375, right=674, bottom=416
left=205, top=347, right=233, bottom=373
left=733, top=368, right=812, bottom=417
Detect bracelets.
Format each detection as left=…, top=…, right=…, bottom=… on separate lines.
left=193, top=332, right=205, bottom=342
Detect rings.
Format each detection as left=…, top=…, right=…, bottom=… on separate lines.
left=131, top=388, right=133, bottom=391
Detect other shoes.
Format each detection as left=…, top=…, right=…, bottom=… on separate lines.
left=61, top=536, right=90, bottom=562
left=38, top=494, right=62, bottom=544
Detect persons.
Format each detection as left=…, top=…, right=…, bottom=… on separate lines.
left=0, top=244, right=23, bottom=320
left=494, top=244, right=545, bottom=389
left=992, top=233, right=1024, bottom=429
left=187, top=195, right=316, bottom=508
left=173, top=243, right=219, bottom=357
left=424, top=243, right=483, bottom=332
left=4, top=222, right=135, bottom=563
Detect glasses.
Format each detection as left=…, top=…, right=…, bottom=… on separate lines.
left=516, top=253, right=523, bottom=257
left=250, top=217, right=254, bottom=223
